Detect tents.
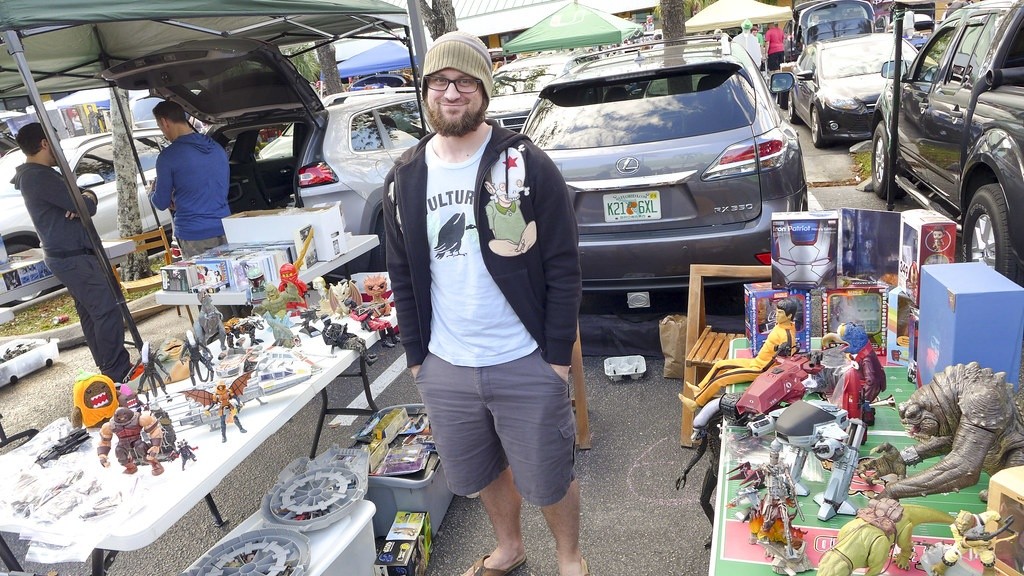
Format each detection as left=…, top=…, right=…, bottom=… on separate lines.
left=503, top=0, right=644, bottom=62
left=320, top=39, right=418, bottom=97
left=0, top=0, right=424, bottom=358
left=685, top=0, right=793, bottom=40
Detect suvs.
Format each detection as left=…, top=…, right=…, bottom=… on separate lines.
left=768, top=32, right=920, bottom=149
left=482, top=47, right=594, bottom=138
left=91, top=30, right=439, bottom=295
left=521, top=32, right=808, bottom=320
left=870, top=0, right=1024, bottom=287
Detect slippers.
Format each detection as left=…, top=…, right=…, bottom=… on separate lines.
left=582, top=559, right=590, bottom=576
left=462, top=552, right=527, bottom=576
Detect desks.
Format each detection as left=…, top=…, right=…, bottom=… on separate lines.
left=0, top=306, right=397, bottom=576
left=155, top=235, right=381, bottom=317
left=0, top=245, right=128, bottom=306
left=708, top=337, right=991, bottom=576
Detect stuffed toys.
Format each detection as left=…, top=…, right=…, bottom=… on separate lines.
left=70, top=370, right=121, bottom=429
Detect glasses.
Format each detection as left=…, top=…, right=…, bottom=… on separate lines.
left=425, top=75, right=482, bottom=93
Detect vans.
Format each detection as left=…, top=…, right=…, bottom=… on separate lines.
left=782, top=0, right=936, bottom=63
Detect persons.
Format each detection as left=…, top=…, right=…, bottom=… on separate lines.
left=146, top=101, right=230, bottom=257
left=677, top=300, right=796, bottom=411
left=245, top=267, right=271, bottom=308
left=383, top=30, right=590, bottom=576
left=278, top=263, right=308, bottom=316
left=903, top=6, right=915, bottom=38
left=346, top=300, right=400, bottom=348
left=653, top=34, right=664, bottom=48
left=98, top=407, right=164, bottom=474
left=11, top=124, right=135, bottom=388
left=319, top=314, right=378, bottom=366
left=752, top=27, right=764, bottom=45
left=765, top=22, right=786, bottom=69
left=942, top=0, right=969, bottom=20
left=211, top=380, right=247, bottom=443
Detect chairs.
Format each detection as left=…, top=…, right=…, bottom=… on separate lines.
left=697, top=75, right=722, bottom=92
left=372, top=115, right=397, bottom=129
left=103, top=225, right=195, bottom=325
left=679, top=264, right=771, bottom=448
left=602, top=86, right=629, bottom=103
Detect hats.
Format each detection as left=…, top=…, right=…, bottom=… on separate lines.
left=420, top=31, right=493, bottom=111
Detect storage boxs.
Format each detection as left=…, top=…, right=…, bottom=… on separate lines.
left=371, top=510, right=434, bottom=576
left=182, top=498, right=378, bottom=576
left=0, top=338, right=60, bottom=388
left=347, top=403, right=453, bottom=538
left=221, top=204, right=348, bottom=271
left=102, top=240, right=136, bottom=258
left=741, top=208, right=1024, bottom=394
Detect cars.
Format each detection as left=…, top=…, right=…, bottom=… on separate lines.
left=0, top=86, right=215, bottom=312
